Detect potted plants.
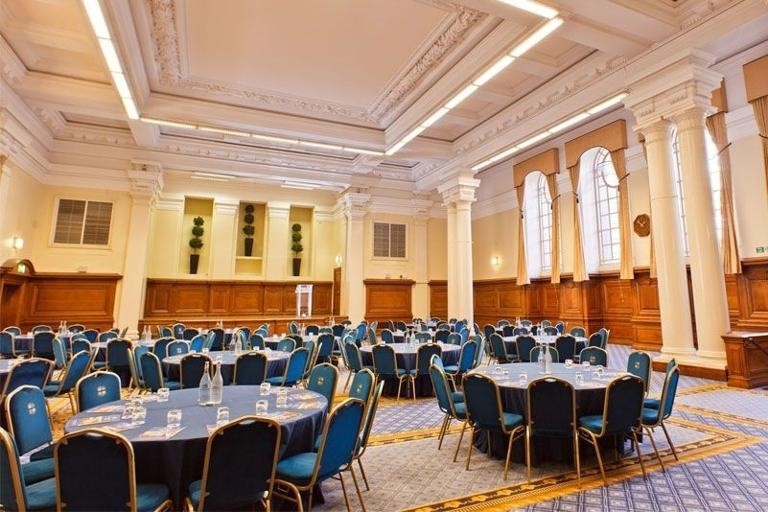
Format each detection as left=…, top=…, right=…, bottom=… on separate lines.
left=190, top=217, right=206, bottom=274
left=292, top=223, right=303, bottom=276
left=244, top=204, right=254, bottom=256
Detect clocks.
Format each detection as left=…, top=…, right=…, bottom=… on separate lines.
left=632, top=214, right=651, bottom=238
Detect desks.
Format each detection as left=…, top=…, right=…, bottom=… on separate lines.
left=65, top=384, right=328, bottom=474
left=90, top=340, right=154, bottom=361
left=360, top=343, right=461, bottom=366
left=162, top=351, right=290, bottom=377
left=14, top=334, right=73, bottom=352
left=264, top=336, right=317, bottom=349
left=466, top=363, right=632, bottom=416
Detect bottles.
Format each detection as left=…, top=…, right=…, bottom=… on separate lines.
left=210, top=362, right=222, bottom=404
left=229, top=331, right=235, bottom=352
left=198, top=362, right=211, bottom=406
left=536, top=322, right=540, bottom=338
left=539, top=322, right=545, bottom=340
left=405, top=328, right=410, bottom=348
left=537, top=342, right=545, bottom=374
left=517, top=317, right=520, bottom=327
left=413, top=320, right=416, bottom=331
left=296, top=323, right=301, bottom=336
left=140, top=325, right=146, bottom=344
left=235, top=333, right=244, bottom=354
left=62, top=321, right=67, bottom=334
left=219, top=320, right=224, bottom=328
left=416, top=320, right=421, bottom=332
left=514, top=316, right=517, bottom=327
left=545, top=342, right=551, bottom=375
left=410, top=328, right=414, bottom=348
left=331, top=316, right=335, bottom=327
left=328, top=316, right=331, bottom=327
left=58, top=321, right=63, bottom=334
left=216, top=320, right=219, bottom=328
left=301, top=323, right=305, bottom=337
left=146, top=326, right=152, bottom=344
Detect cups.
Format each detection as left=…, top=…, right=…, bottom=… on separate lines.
left=415, top=339, right=419, bottom=345
left=597, top=365, right=603, bottom=373
left=166, top=409, right=181, bottom=427
left=256, top=400, right=268, bottom=417
left=495, top=366, right=502, bottom=376
left=156, top=387, right=170, bottom=402
left=582, top=361, right=590, bottom=372
left=259, top=382, right=271, bottom=396
left=176, top=348, right=181, bottom=354
left=592, top=370, right=600, bottom=381
left=276, top=389, right=287, bottom=408
left=216, top=355, right=222, bottom=363
left=202, top=348, right=207, bottom=355
left=504, top=369, right=509, bottom=379
left=576, top=372, right=584, bottom=386
left=217, top=407, right=229, bottom=426
left=131, top=405, right=146, bottom=424
left=254, top=345, right=258, bottom=353
left=518, top=372, right=528, bottom=386
left=188, top=349, right=197, bottom=355
left=564, top=359, right=572, bottom=368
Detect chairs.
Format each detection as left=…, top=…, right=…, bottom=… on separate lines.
left=445, top=343, right=475, bottom=390
left=251, top=325, right=269, bottom=350
left=0, top=332, right=13, bottom=356
left=286, top=321, right=352, bottom=335
left=489, top=334, right=510, bottom=363
left=235, top=352, right=266, bottom=384
left=78, top=373, right=122, bottom=410
left=4, top=386, right=58, bottom=460
left=127, top=346, right=180, bottom=394
left=579, top=347, right=607, bottom=367
left=526, top=377, right=577, bottom=481
left=430, top=364, right=466, bottom=450
left=349, top=380, right=385, bottom=490
left=348, top=366, right=374, bottom=405
left=0, top=325, right=86, bottom=335
left=157, top=324, right=199, bottom=340
left=555, top=337, right=575, bottom=362
left=1, top=358, right=53, bottom=402
left=186, top=415, right=281, bottom=512
left=1, top=427, right=57, bottom=512
left=310, top=363, right=339, bottom=410
left=208, top=326, right=250, bottom=351
left=643, top=366, right=679, bottom=472
left=156, top=337, right=206, bottom=356
left=517, top=336, right=536, bottom=361
left=269, top=350, right=309, bottom=388
left=53, top=328, right=118, bottom=370
left=181, top=354, right=213, bottom=389
left=484, top=320, right=585, bottom=336
left=344, top=342, right=361, bottom=392
left=589, top=328, right=610, bottom=348
left=388, top=317, right=468, bottom=330
left=319, top=336, right=333, bottom=362
left=58, top=430, right=171, bottom=512
left=578, top=373, right=647, bottom=488
left=454, top=372, right=524, bottom=471
left=372, top=345, right=407, bottom=400
left=340, top=329, right=483, bottom=344
left=627, top=353, right=651, bottom=394
left=410, top=344, right=442, bottom=400
left=35, top=333, right=55, bottom=357
left=277, top=399, right=366, bottom=512
